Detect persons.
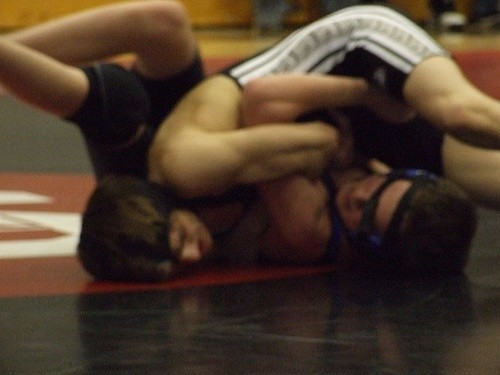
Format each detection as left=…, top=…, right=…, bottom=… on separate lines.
left=73, top=3, right=500, bottom=285
left=0, top=0, right=483, bottom=285
